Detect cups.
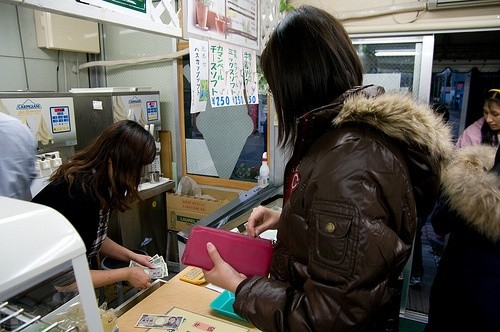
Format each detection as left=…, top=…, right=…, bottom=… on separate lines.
left=148, top=171, right=160, bottom=184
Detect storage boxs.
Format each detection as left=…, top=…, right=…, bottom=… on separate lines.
left=166, top=175, right=258, bottom=232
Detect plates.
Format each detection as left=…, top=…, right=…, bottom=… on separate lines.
left=209, top=289, right=247, bottom=323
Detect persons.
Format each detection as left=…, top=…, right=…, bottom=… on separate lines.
left=409, top=88, right=500, bottom=332
left=202, top=6, right=417, bottom=332
left=0, top=109, right=157, bottom=292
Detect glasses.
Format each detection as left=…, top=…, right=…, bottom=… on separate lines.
left=266, top=87, right=273, bottom=96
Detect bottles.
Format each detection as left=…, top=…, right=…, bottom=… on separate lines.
left=259, top=158, right=270, bottom=186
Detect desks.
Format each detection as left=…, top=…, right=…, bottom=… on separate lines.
left=117, top=266, right=263, bottom=332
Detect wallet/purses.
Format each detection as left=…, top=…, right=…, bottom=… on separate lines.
left=181, top=224, right=275, bottom=279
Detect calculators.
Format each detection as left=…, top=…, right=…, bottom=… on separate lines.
left=179, top=265, right=207, bottom=285
